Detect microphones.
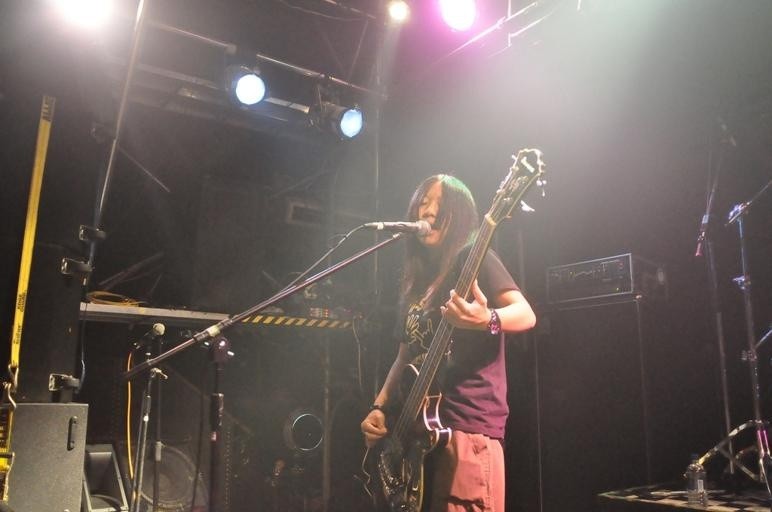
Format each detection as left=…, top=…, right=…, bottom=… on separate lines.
left=366, top=220, right=432, bottom=238
left=713, top=113, right=738, bottom=152
left=127, top=322, right=173, bottom=351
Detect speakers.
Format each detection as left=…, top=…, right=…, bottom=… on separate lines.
left=118, top=332, right=211, bottom=512
left=216, top=329, right=331, bottom=511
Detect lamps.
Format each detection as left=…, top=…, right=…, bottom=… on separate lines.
left=307, top=79, right=366, bottom=142
left=222, top=43, right=272, bottom=110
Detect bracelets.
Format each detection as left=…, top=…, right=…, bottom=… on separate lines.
left=488, top=306, right=501, bottom=336
left=370, top=403, right=385, bottom=413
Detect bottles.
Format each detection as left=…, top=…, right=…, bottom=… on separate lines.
left=684, top=454, right=708, bottom=511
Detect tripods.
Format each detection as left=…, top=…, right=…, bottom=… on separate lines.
left=683, top=147, right=772, bottom=504
